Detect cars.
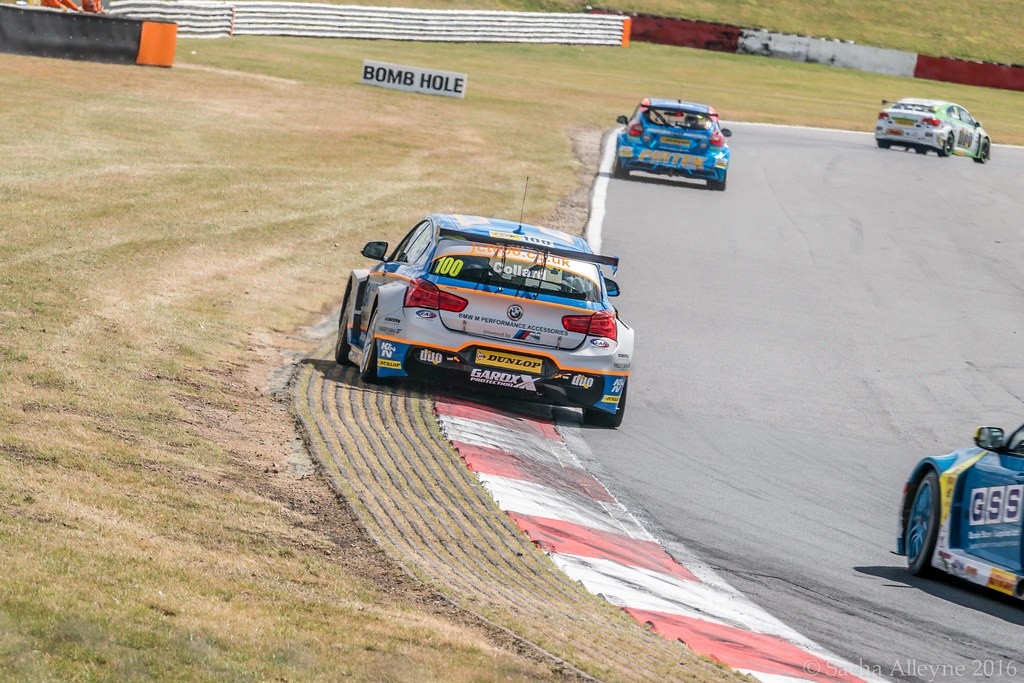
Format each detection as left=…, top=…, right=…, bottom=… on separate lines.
left=875, top=97, right=991, bottom=164
left=891, top=415, right=1024, bottom=610
left=610, top=97, right=732, bottom=192
left=334, top=177, right=638, bottom=429
